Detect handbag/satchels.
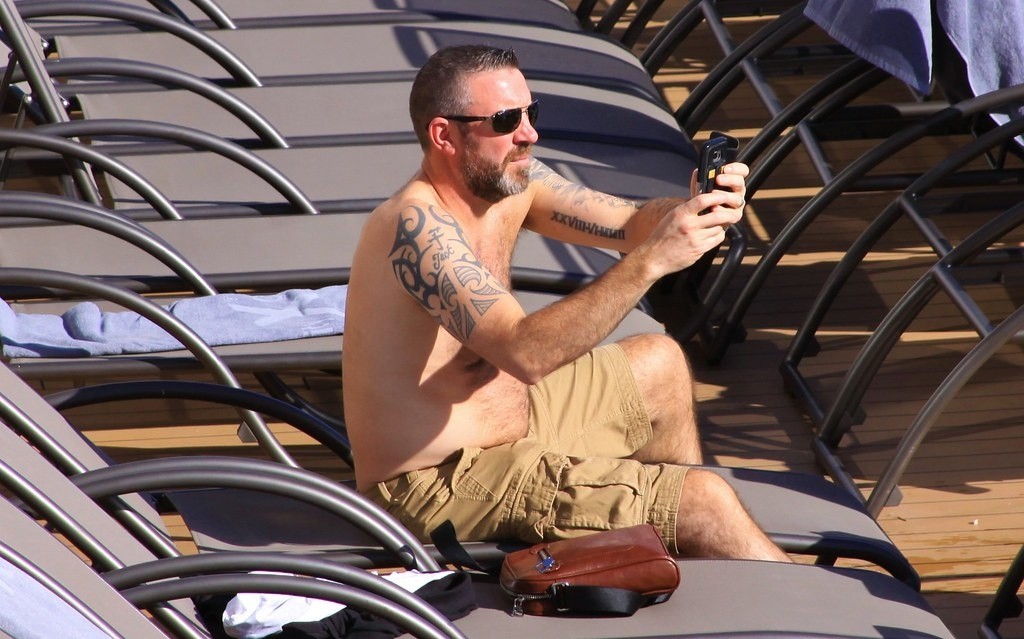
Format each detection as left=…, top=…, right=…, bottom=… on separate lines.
left=430, top=520, right=680, bottom=619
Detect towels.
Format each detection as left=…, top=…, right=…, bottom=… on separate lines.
left=0, top=283, right=348, bottom=359
left=802, top=0, right=1024, bottom=148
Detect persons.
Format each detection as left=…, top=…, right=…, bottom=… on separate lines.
left=341, top=43, right=796, bottom=563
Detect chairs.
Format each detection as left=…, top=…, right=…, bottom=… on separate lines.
left=1, top=0, right=1024, bottom=638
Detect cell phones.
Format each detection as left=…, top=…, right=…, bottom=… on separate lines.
left=695, top=136, right=728, bottom=216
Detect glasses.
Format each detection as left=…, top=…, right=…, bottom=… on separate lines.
left=424, top=99, right=540, bottom=135
